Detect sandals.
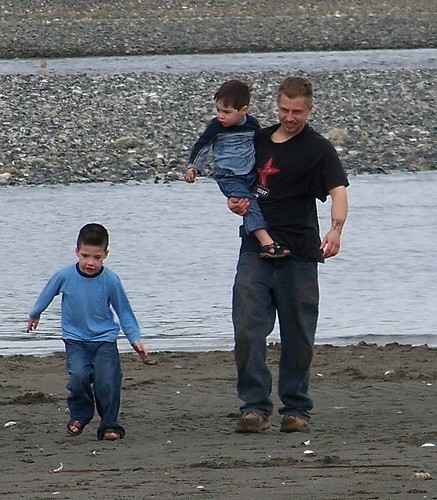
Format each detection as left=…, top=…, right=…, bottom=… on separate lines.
left=104, top=428, right=117, bottom=439
left=68, top=420, right=83, bottom=434
left=260, top=243, right=290, bottom=257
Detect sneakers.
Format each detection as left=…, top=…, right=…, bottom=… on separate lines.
left=235, top=412, right=269, bottom=432
left=280, top=416, right=308, bottom=433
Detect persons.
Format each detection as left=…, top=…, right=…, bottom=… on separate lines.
left=27, top=223, right=147, bottom=441
left=227, top=77, right=350, bottom=432
left=185, top=80, right=291, bottom=258
